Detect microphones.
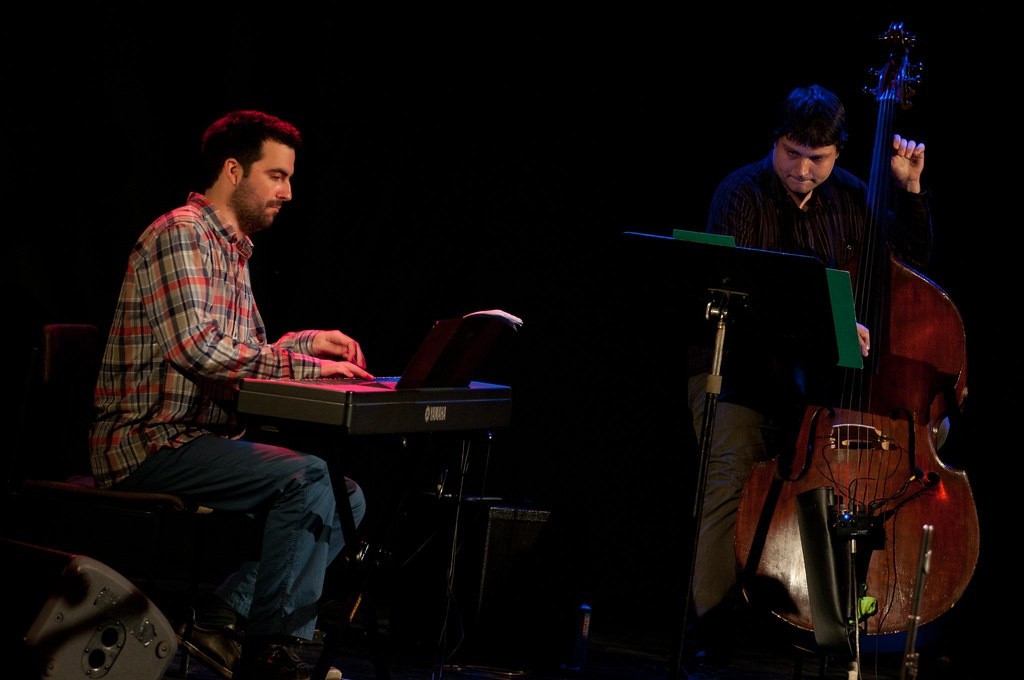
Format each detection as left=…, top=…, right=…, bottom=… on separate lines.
left=924, top=472, right=942, bottom=487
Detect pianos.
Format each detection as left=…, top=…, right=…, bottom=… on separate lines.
left=237, top=307, right=541, bottom=427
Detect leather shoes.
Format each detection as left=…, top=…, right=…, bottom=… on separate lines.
left=172, top=607, right=242, bottom=680
left=232, top=638, right=350, bottom=680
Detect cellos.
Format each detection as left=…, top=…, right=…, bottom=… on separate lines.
left=734, top=24, right=981, bottom=639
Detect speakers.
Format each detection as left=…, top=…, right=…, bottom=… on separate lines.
left=0, top=536, right=179, bottom=680
left=477, top=504, right=551, bottom=620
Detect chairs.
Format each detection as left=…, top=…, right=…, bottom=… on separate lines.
left=0, top=320, right=216, bottom=680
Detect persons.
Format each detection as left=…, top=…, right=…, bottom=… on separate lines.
left=90, top=110, right=377, bottom=680
left=690, top=85, right=937, bottom=619
